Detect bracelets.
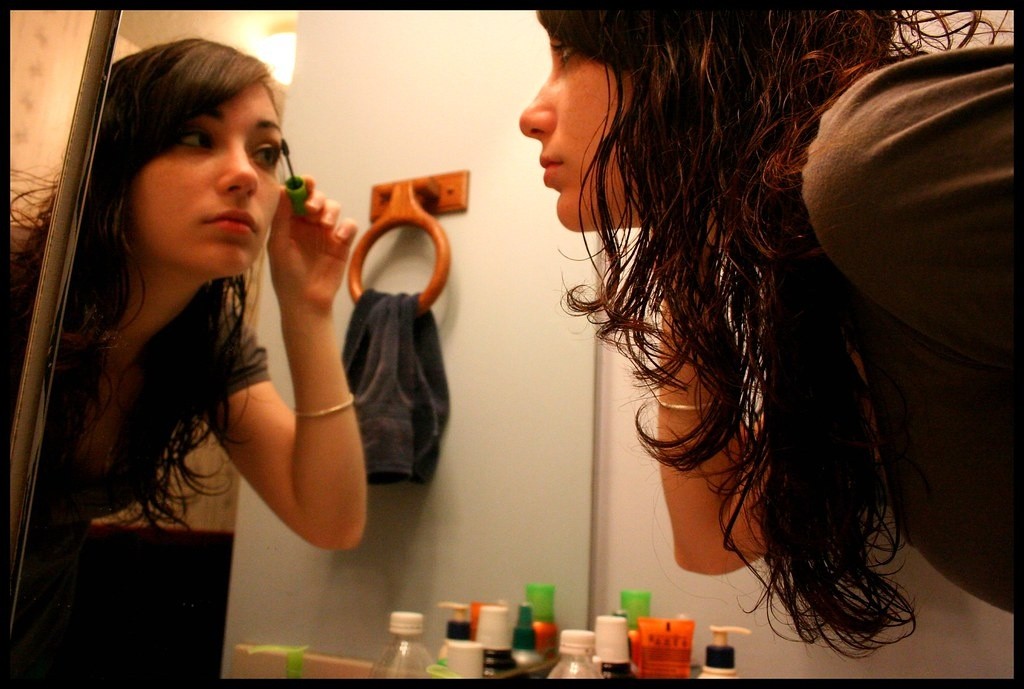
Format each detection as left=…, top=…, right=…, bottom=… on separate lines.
left=293, top=393, right=355, bottom=418
left=656, top=399, right=718, bottom=412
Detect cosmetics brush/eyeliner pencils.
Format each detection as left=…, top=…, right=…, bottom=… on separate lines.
left=278, top=139, right=308, bottom=214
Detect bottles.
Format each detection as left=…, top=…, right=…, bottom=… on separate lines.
left=595, top=614, right=636, bottom=679
left=474, top=605, right=517, bottom=672
left=547, top=628, right=603, bottom=679
left=511, top=602, right=543, bottom=666
left=367, top=611, right=433, bottom=679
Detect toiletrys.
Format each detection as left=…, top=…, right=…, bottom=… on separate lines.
left=246, top=576, right=558, bottom=678
left=550, top=591, right=753, bottom=680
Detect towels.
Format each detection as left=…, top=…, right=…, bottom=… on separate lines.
left=338, top=283, right=449, bottom=487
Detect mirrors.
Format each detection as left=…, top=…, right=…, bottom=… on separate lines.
left=10, top=10, right=600, bottom=679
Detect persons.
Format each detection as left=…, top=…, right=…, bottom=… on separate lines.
left=10, top=39, right=370, bottom=688
left=520, top=8, right=1016, bottom=678
